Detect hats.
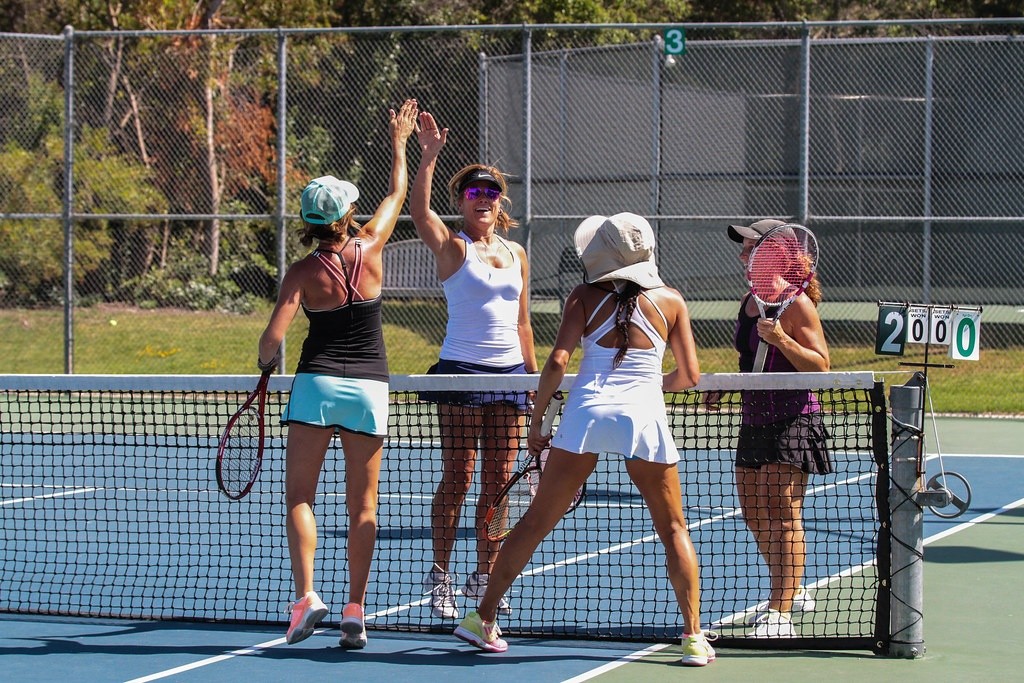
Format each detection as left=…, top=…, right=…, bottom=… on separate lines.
left=574, top=211, right=665, bottom=289
left=302, top=175, right=359, bottom=224
left=458, top=170, right=503, bottom=192
left=728, top=219, right=797, bottom=247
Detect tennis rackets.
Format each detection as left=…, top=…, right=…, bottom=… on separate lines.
left=484, top=392, right=565, bottom=543
left=747, top=223, right=820, bottom=370
left=523, top=429, right=587, bottom=513
left=215, top=373, right=271, bottom=501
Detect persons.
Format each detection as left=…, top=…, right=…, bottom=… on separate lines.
left=453, top=212, right=716, bottom=665
left=408, top=111, right=538, bottom=621
left=259, top=100, right=419, bottom=650
left=701, top=220, right=833, bottom=638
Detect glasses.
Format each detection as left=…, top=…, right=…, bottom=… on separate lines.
left=465, top=184, right=500, bottom=200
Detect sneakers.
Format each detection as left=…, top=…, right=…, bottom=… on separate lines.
left=339, top=603, right=367, bottom=649
left=462, top=572, right=513, bottom=617
left=747, top=608, right=796, bottom=636
left=681, top=631, right=718, bottom=666
left=287, top=591, right=329, bottom=645
left=748, top=585, right=815, bottom=626
left=454, top=612, right=508, bottom=652
left=426, top=568, right=459, bottom=620
